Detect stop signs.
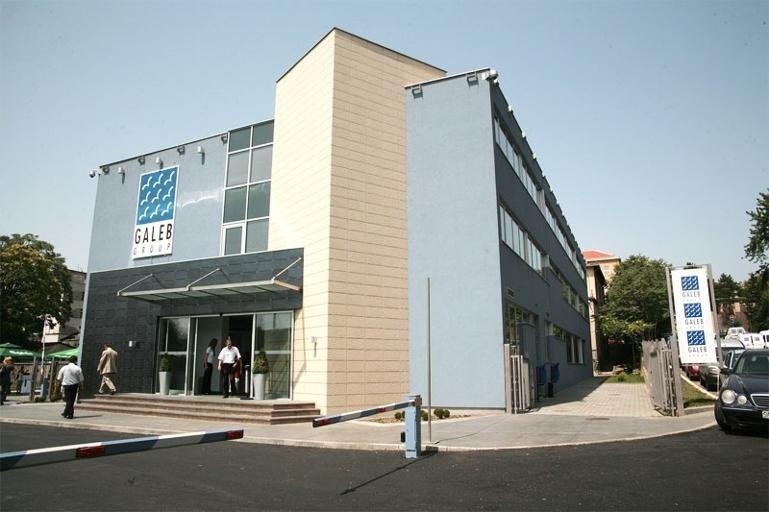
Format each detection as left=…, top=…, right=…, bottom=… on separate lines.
left=729, top=314, right=736, bottom=320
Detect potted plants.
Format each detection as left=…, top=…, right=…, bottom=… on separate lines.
left=158, top=352, right=172, bottom=395
left=252, top=349, right=270, bottom=401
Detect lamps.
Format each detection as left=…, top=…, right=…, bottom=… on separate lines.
left=155, top=157, right=163, bottom=165
left=118, top=167, right=125, bottom=175
left=197, top=146, right=205, bottom=155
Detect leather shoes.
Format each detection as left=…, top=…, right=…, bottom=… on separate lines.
left=61, top=413, right=73, bottom=418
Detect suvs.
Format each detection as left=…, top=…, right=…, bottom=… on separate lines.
left=712, top=347, right=769, bottom=437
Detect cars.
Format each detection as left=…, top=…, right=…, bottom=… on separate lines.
left=669, top=325, right=769, bottom=392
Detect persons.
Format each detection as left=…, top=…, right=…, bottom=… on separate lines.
left=0, top=356, right=30, bottom=405
left=201, top=338, right=217, bottom=395
left=97, top=343, right=119, bottom=395
left=217, top=336, right=242, bottom=398
left=592, top=359, right=601, bottom=376
left=228, top=358, right=242, bottom=394
left=56, top=356, right=85, bottom=419
